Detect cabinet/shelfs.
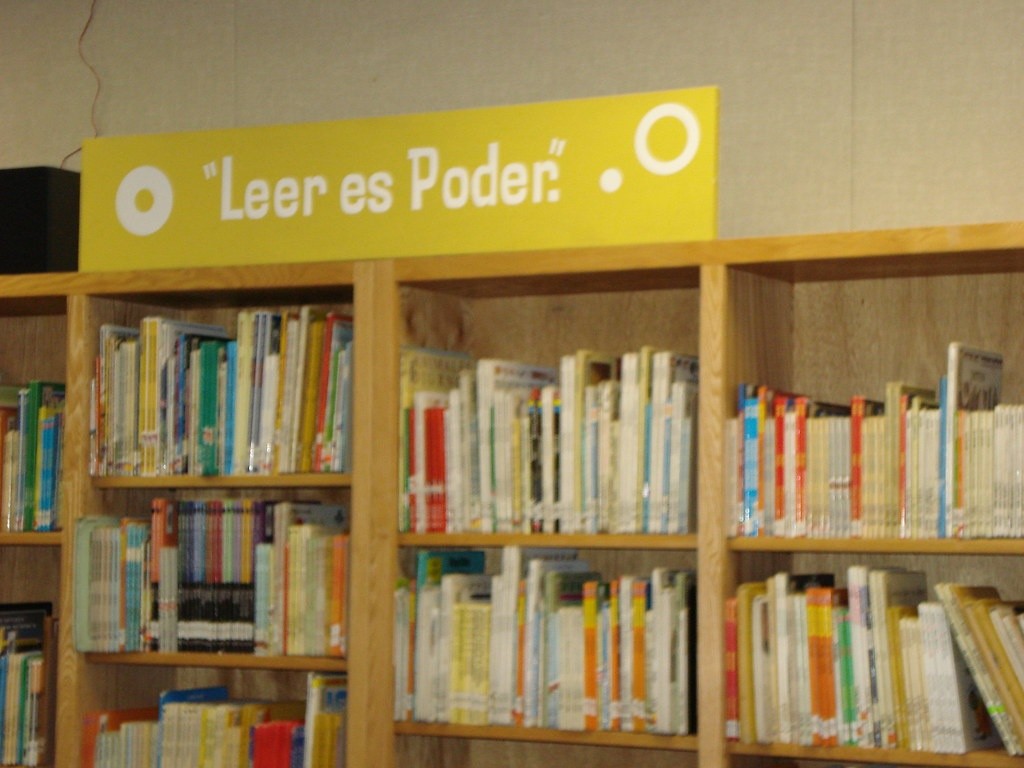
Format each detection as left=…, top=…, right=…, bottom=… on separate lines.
left=372, top=222, right=1024, bottom=768
left=1, top=258, right=371, bottom=768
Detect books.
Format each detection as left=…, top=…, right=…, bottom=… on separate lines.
left=0, top=300, right=1024, bottom=768
